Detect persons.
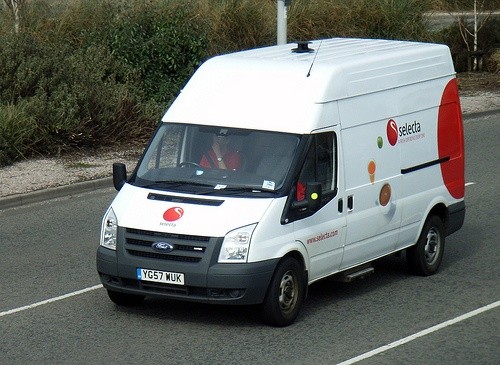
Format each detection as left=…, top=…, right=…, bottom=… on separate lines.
left=199, top=131, right=241, bottom=174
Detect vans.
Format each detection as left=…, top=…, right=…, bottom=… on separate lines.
left=96, top=37, right=465, bottom=327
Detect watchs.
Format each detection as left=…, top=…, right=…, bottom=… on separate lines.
left=216, top=157, right=223, bottom=162
